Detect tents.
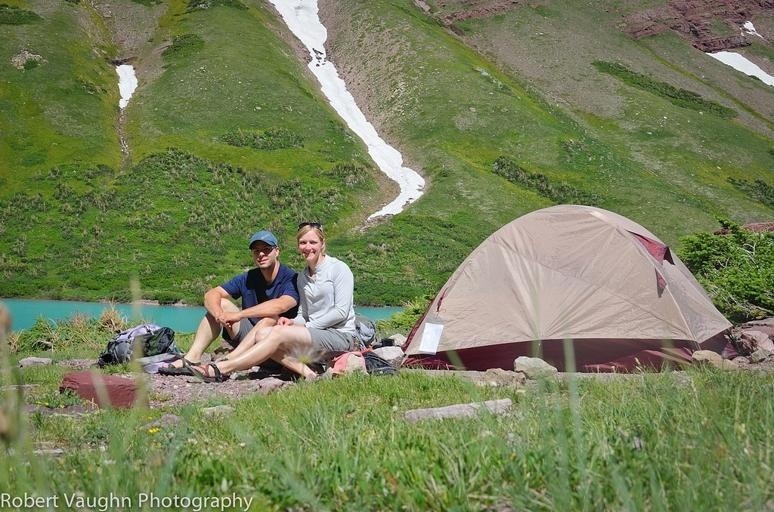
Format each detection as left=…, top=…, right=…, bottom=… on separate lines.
left=400, top=204, right=739, bottom=371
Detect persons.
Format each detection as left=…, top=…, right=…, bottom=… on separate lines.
left=158, top=222, right=360, bottom=384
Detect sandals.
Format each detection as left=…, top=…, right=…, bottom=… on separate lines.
left=158, top=357, right=230, bottom=383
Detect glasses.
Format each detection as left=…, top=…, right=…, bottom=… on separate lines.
left=298, top=222, right=322, bottom=231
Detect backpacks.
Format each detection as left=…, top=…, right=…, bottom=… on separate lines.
left=354, top=316, right=376, bottom=345
left=97, top=324, right=184, bottom=374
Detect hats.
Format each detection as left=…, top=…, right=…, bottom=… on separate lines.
left=249, top=231, right=278, bottom=249
